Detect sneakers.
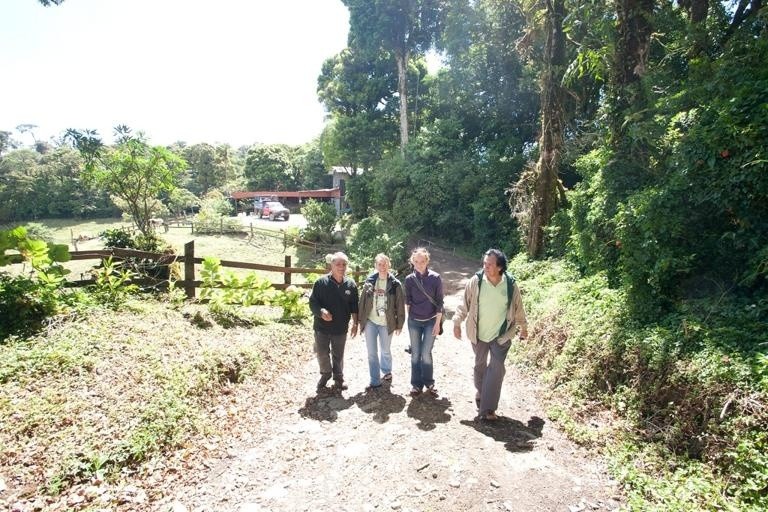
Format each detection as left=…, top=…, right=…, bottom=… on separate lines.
left=476, top=392, right=499, bottom=421
left=366, top=372, right=392, bottom=391
left=317, top=374, right=343, bottom=388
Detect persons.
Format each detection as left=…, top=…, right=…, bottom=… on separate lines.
left=357, top=253, right=405, bottom=390
left=404, top=246, right=444, bottom=395
left=307, top=251, right=359, bottom=391
left=453, top=248, right=527, bottom=423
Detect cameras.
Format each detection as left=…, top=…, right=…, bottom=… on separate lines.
left=439, top=320, right=445, bottom=335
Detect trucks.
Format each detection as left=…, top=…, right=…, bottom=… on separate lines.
left=254, top=196, right=289, bottom=221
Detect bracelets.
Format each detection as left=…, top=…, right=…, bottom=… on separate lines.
left=352, top=323, right=357, bottom=327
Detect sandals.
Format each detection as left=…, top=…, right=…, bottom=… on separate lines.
left=410, top=383, right=438, bottom=394
